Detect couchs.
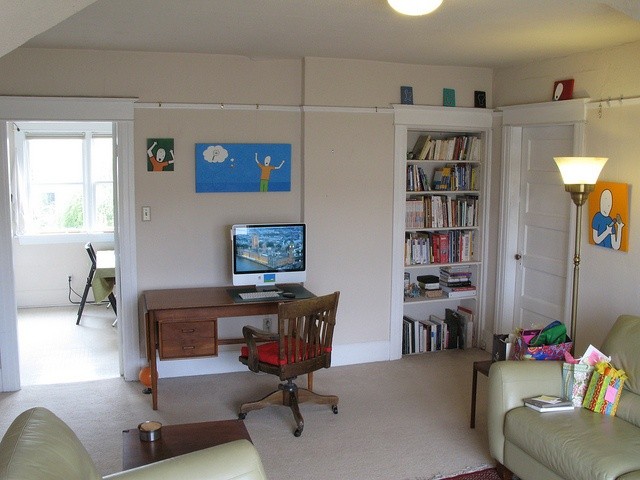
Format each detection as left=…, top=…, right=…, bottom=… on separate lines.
left=1, top=407, right=269, bottom=477
left=487, top=313, right=640, bottom=479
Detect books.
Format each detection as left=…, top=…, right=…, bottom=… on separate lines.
left=405, top=229, right=475, bottom=267
left=417, top=265, right=477, bottom=302
left=405, top=194, right=479, bottom=229
left=403, top=306, right=475, bottom=356
left=411, top=134, right=481, bottom=161
left=521, top=394, right=574, bottom=413
left=407, top=163, right=479, bottom=192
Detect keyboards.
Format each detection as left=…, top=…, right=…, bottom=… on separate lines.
left=238, top=291, right=279, bottom=300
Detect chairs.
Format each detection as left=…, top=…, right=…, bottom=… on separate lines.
left=239, top=291, right=342, bottom=438
left=76, top=241, right=117, bottom=324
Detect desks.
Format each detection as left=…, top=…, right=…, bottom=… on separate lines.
left=121, top=420, right=254, bottom=471
left=140, top=283, right=319, bottom=411
left=91, top=250, right=115, bottom=301
left=469, top=359, right=501, bottom=427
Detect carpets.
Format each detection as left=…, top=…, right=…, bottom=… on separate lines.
left=436, top=465, right=519, bottom=480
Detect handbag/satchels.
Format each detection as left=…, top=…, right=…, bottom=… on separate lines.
left=492, top=333, right=518, bottom=363
left=582, top=363, right=628, bottom=416
left=561, top=358, right=595, bottom=407
left=512, top=329, right=573, bottom=360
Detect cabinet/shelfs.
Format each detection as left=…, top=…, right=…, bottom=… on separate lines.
left=394, top=103, right=494, bottom=356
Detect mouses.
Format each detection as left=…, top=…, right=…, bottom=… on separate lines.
left=283, top=292, right=295, bottom=298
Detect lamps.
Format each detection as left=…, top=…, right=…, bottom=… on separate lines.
left=389, top=0, right=444, bottom=18
left=553, top=156, right=608, bottom=363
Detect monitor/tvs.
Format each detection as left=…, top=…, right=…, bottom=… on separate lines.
left=231, top=223, right=306, bottom=293
